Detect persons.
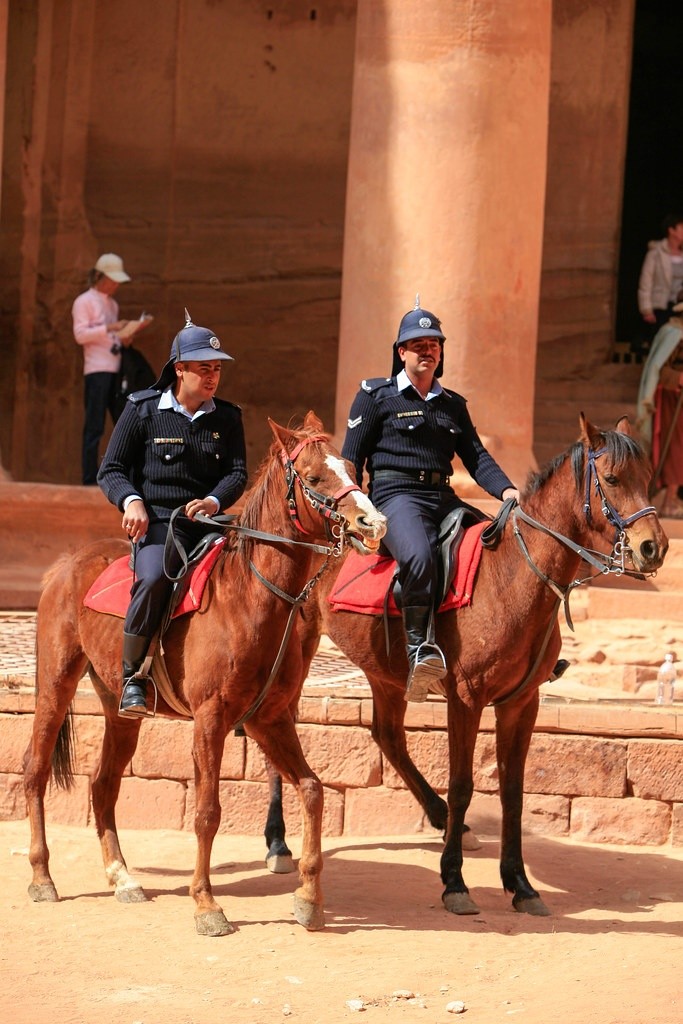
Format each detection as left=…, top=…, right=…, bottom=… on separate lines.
left=72, top=252, right=133, bottom=488
left=340, top=296, right=522, bottom=703
left=95, top=307, right=249, bottom=719
left=636, top=214, right=683, bottom=321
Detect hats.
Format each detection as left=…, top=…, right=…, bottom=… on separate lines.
left=390, top=293, right=446, bottom=378
left=94, top=252, right=129, bottom=282
left=150, top=308, right=234, bottom=391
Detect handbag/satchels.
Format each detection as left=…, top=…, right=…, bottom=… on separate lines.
left=112, top=343, right=159, bottom=404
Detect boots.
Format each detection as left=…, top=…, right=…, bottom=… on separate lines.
left=119, top=630, right=153, bottom=718
left=401, top=604, right=447, bottom=702
left=546, top=658, right=572, bottom=681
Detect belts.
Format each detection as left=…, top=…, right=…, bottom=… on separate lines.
left=369, top=467, right=450, bottom=487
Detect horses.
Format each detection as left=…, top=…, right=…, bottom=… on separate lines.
left=22, top=410, right=386, bottom=936
left=260, top=409, right=668, bottom=914
